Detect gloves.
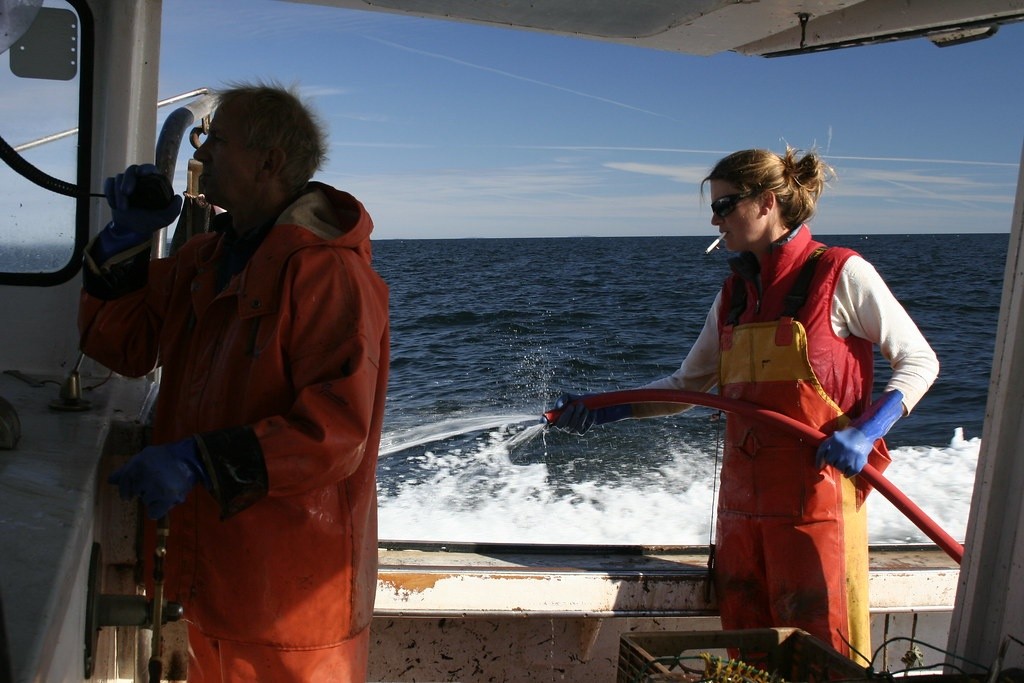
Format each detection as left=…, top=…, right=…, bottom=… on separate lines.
left=817, top=390, right=905, bottom=478
left=99, top=163, right=183, bottom=256
left=540, top=391, right=632, bottom=436
left=108, top=436, right=213, bottom=519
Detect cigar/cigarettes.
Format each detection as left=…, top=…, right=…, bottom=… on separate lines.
left=705, top=232, right=728, bottom=254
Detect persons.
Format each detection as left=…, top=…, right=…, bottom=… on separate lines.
left=556, top=148, right=940, bottom=683
left=76, top=85, right=390, bottom=683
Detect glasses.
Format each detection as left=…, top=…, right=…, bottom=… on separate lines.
left=710, top=187, right=766, bottom=217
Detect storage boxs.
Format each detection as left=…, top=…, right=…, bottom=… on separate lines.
left=616, top=627, right=879, bottom=683
